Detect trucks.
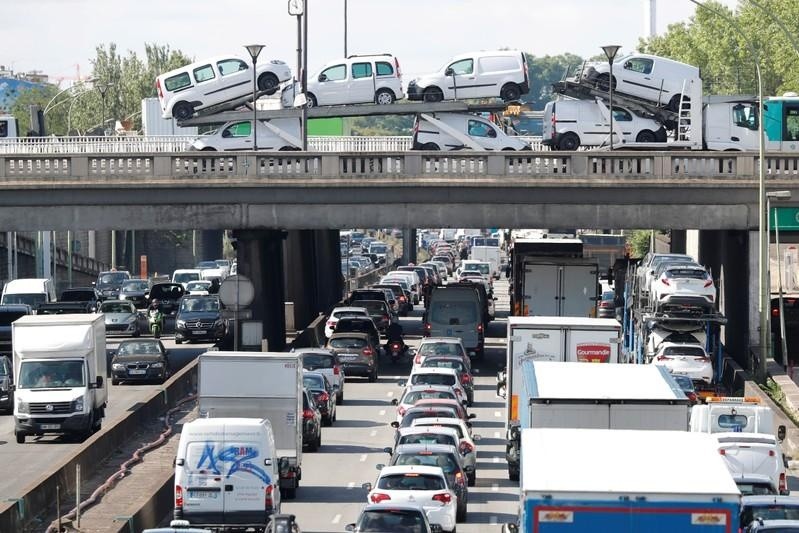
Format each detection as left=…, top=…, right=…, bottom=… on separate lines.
left=194, top=350, right=305, bottom=501
left=500, top=360, right=693, bottom=482
left=501, top=428, right=744, bottom=533
left=495, top=317, right=625, bottom=400
left=8, top=313, right=113, bottom=443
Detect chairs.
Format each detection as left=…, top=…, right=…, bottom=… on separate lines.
left=437, top=457, right=448, bottom=466
left=406, top=437, right=419, bottom=444
left=401, top=516, right=417, bottom=526
left=437, top=438, right=448, bottom=443
left=367, top=514, right=385, bottom=529
left=407, top=456, right=420, bottom=465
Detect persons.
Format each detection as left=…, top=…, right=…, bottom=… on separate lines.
left=383, top=317, right=409, bottom=357
left=147, top=299, right=166, bottom=323
left=61, top=363, right=82, bottom=382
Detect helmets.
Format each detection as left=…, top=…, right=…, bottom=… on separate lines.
left=152, top=299, right=159, bottom=306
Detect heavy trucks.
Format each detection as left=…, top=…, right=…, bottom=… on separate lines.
left=140, top=93, right=799, bottom=170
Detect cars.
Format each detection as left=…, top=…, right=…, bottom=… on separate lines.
left=0, top=227, right=745, bottom=532
left=301, top=372, right=340, bottom=427
left=302, top=387, right=323, bottom=452
left=737, top=474, right=799, bottom=532
left=107, top=338, right=171, bottom=387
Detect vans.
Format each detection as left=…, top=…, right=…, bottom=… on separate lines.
left=717, top=425, right=794, bottom=495
left=173, top=415, right=290, bottom=529
left=692, top=396, right=776, bottom=435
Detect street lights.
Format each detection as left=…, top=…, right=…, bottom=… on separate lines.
left=765, top=190, right=792, bottom=359
left=43, top=76, right=116, bottom=138
left=97, top=83, right=109, bottom=135
left=247, top=45, right=267, bottom=148
left=600, top=45, right=622, bottom=150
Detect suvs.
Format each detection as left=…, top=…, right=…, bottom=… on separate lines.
left=408, top=50, right=536, bottom=103
left=279, top=53, right=407, bottom=108
left=541, top=98, right=669, bottom=151
left=574, top=54, right=704, bottom=116
left=412, top=113, right=535, bottom=154
left=189, top=118, right=302, bottom=152
left=155, top=55, right=291, bottom=120
